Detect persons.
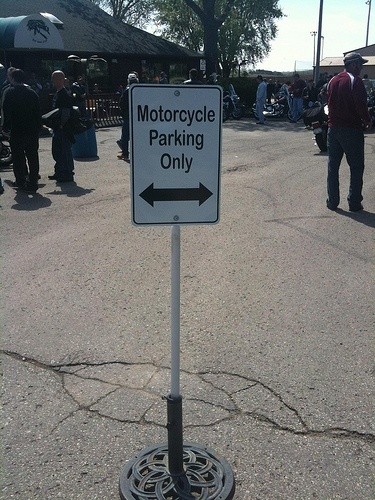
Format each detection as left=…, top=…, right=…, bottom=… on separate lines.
left=118, top=71, right=139, bottom=160
left=73, top=77, right=87, bottom=111
left=0, top=67, right=44, bottom=194
left=256, top=74, right=337, bottom=151
left=184, top=69, right=203, bottom=85
left=48, top=70, right=75, bottom=182
left=160, top=72, right=167, bottom=84
left=362, top=73, right=375, bottom=112
left=206, top=75, right=222, bottom=85
left=327, top=52, right=368, bottom=211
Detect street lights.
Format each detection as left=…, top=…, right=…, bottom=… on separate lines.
left=310, top=31, right=317, bottom=82
left=321, top=36, right=324, bottom=60
left=365, top=0, right=372, bottom=47
left=65, top=54, right=107, bottom=111
left=234, top=60, right=246, bottom=77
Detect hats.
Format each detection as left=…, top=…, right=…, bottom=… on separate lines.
left=343, top=52, right=369, bottom=65
left=127, top=73, right=137, bottom=80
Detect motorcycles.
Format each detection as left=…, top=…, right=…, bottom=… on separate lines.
left=311, top=85, right=375, bottom=152
left=252, top=93, right=302, bottom=121
left=223, top=83, right=243, bottom=121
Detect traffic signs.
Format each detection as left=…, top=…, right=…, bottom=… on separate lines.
left=128, top=83, right=223, bottom=225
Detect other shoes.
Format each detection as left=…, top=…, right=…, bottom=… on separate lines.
left=290, top=120, right=297, bottom=123
left=348, top=203, right=364, bottom=212
left=326, top=201, right=338, bottom=211
left=47, top=173, right=74, bottom=183
left=117, top=140, right=122, bottom=150
left=256, top=122, right=265, bottom=125
left=117, top=155, right=130, bottom=163
left=304, top=125, right=311, bottom=130
left=14, top=179, right=38, bottom=193
